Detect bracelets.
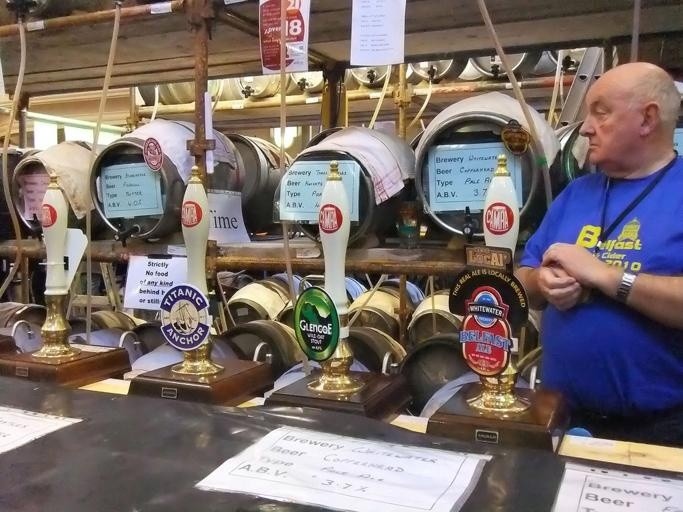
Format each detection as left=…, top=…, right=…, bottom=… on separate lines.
left=616, top=272, right=635, bottom=301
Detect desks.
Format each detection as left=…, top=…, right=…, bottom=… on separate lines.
left=1, top=374, right=683, bottom=512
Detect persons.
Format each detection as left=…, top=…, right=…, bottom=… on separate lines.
left=512, top=62, right=683, bottom=450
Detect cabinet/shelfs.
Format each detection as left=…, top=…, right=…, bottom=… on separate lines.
left=0, top=1, right=682, bottom=278
